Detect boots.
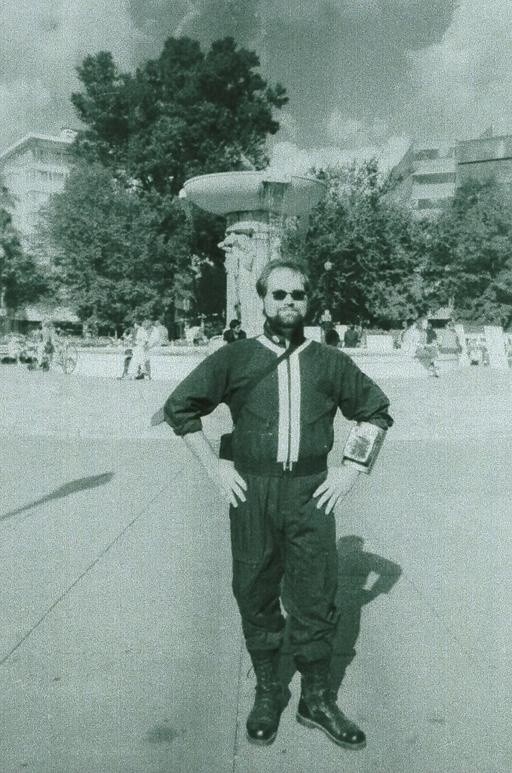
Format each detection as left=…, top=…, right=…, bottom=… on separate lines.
left=293, top=661, right=366, bottom=751
left=244, top=654, right=293, bottom=748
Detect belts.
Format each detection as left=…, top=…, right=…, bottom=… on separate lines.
left=255, top=456, right=327, bottom=478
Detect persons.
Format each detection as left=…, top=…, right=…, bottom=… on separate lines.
left=409, top=323, right=442, bottom=379
left=342, top=324, right=358, bottom=347
left=124, top=319, right=160, bottom=380
left=325, top=324, right=341, bottom=346
left=222, top=319, right=246, bottom=345
left=163, top=259, right=395, bottom=750
left=117, top=320, right=140, bottom=378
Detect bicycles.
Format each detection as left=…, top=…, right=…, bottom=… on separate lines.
left=51, top=327, right=78, bottom=375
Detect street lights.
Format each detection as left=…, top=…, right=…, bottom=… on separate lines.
left=324, top=262, right=333, bottom=309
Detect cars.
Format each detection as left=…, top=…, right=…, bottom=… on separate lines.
left=1, top=332, right=32, bottom=363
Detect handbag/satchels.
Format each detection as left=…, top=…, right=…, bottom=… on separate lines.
left=219, top=432, right=234, bottom=461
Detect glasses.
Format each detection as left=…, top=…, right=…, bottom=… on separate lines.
left=270, top=289, right=306, bottom=301
